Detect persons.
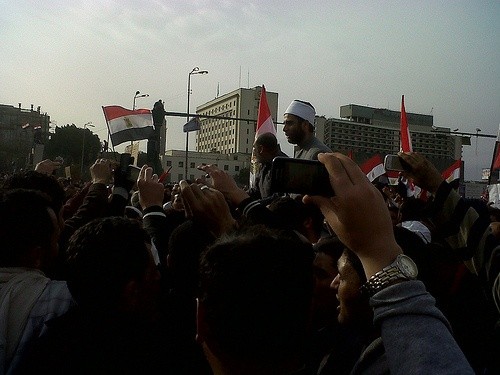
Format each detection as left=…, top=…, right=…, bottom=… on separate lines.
left=282, top=99, right=335, bottom=199
left=0, top=132, right=500, bottom=375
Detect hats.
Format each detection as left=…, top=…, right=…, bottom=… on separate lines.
left=396, top=220, right=431, bottom=244
left=284, top=101, right=315, bottom=126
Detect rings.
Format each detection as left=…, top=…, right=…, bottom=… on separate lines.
left=200, top=185, right=209, bottom=191
left=100, top=159, right=106, bottom=162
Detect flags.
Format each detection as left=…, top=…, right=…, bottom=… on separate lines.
left=248, top=87, right=278, bottom=188
left=346, top=98, right=460, bottom=202
left=103, top=105, right=156, bottom=147
left=489, top=122, right=500, bottom=184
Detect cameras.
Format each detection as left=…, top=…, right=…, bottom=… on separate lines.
left=384, top=154, right=410, bottom=172
left=126, top=164, right=142, bottom=182
left=267, top=156, right=336, bottom=202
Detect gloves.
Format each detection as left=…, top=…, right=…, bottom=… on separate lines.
left=114, top=166, right=134, bottom=194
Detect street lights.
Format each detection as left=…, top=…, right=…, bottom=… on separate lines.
left=80, top=123, right=95, bottom=181
left=184, top=67, right=208, bottom=181
left=131, top=91, right=149, bottom=157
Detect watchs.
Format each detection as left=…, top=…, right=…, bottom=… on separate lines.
left=360, top=253, right=418, bottom=299
